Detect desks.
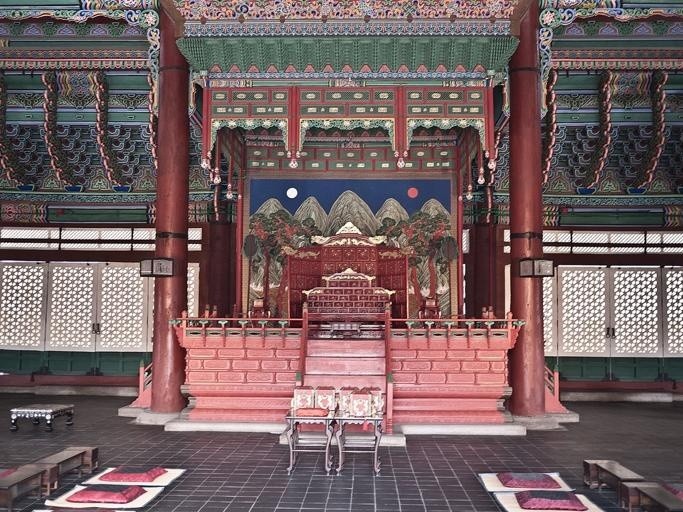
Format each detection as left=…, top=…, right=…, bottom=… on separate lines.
left=334, top=409, right=384, bottom=473
left=0, top=446, right=99, bottom=512
left=284, top=409, right=336, bottom=475
left=583, top=459, right=683, bottom=512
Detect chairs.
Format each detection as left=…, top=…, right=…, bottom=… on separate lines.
left=301, top=265, right=397, bottom=322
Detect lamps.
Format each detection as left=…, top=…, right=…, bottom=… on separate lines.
left=139, top=257, right=174, bottom=278
left=518, top=257, right=554, bottom=277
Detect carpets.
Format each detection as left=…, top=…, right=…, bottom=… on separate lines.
left=43, top=465, right=189, bottom=509
left=478, top=471, right=606, bottom=512
